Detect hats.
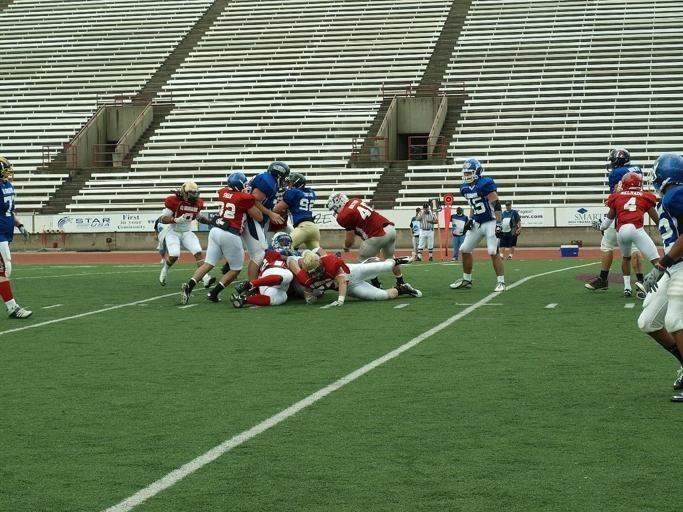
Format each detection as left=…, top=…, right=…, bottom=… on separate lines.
left=423, top=204, right=428, bottom=208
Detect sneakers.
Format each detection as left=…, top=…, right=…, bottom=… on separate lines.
left=204, top=277, right=217, bottom=288
left=493, top=282, right=506, bottom=292
left=6, top=306, right=33, bottom=319
left=637, top=291, right=646, bottom=299
left=401, top=283, right=423, bottom=298
left=233, top=288, right=258, bottom=308
left=231, top=281, right=253, bottom=302
left=180, top=283, right=189, bottom=306
left=672, top=375, right=682, bottom=390
left=449, top=279, right=473, bottom=289
left=159, top=268, right=168, bottom=287
left=394, top=255, right=414, bottom=265
left=207, top=291, right=222, bottom=302
left=584, top=276, right=608, bottom=290
left=635, top=284, right=644, bottom=299
left=624, top=288, right=633, bottom=297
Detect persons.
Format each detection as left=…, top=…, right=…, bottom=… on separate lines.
left=154, top=180, right=216, bottom=288
left=287, top=247, right=341, bottom=305
left=300, top=255, right=423, bottom=307
left=242, top=162, right=290, bottom=286
left=407, top=208, right=426, bottom=260
left=271, top=174, right=324, bottom=251
left=451, top=209, right=468, bottom=261
left=499, top=200, right=522, bottom=260
left=587, top=148, right=648, bottom=291
left=228, top=231, right=293, bottom=309
left=0, top=156, right=33, bottom=319
left=637, top=153, right=683, bottom=403
left=155, top=207, right=169, bottom=264
left=180, top=171, right=263, bottom=304
left=326, top=192, right=406, bottom=291
left=450, top=157, right=506, bottom=293
left=601, top=172, right=664, bottom=302
left=416, top=204, right=439, bottom=261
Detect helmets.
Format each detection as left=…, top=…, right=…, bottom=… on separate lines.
left=620, top=172, right=641, bottom=191
left=287, top=174, right=307, bottom=190
left=328, top=192, right=349, bottom=217
left=272, top=231, right=293, bottom=253
left=268, top=161, right=290, bottom=182
left=0, top=157, right=14, bottom=182
left=461, top=158, right=484, bottom=183
left=646, top=152, right=683, bottom=192
left=181, top=181, right=200, bottom=206
left=228, top=172, right=248, bottom=191
left=607, top=148, right=630, bottom=169
left=302, top=254, right=325, bottom=279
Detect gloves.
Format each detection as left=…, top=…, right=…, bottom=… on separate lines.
left=495, top=225, right=504, bottom=240
left=306, top=294, right=318, bottom=304
left=463, top=218, right=474, bottom=234
left=174, top=213, right=186, bottom=223
left=320, top=301, right=343, bottom=309
left=18, top=224, right=30, bottom=241
left=643, top=266, right=664, bottom=294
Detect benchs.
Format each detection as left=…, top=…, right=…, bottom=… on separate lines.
left=448, top=135, right=683, bottom=150
left=0, top=92, right=137, bottom=99
left=466, top=86, right=683, bottom=99
left=13, top=196, right=51, bottom=202
left=0, top=152, right=61, bottom=159
left=8, top=166, right=39, bottom=173
left=12, top=188, right=56, bottom=195
left=167, top=104, right=381, bottom=117
left=0, top=125, right=81, bottom=131
left=7, top=160, right=52, bottom=165
left=405, top=168, right=654, bottom=180
left=128, top=159, right=348, bottom=171
left=166, top=1, right=452, bottom=84
left=465, top=93, right=683, bottom=105
left=154, top=130, right=369, bottom=141
left=402, top=176, right=653, bottom=189
left=452, top=122, right=682, bottom=134
left=157, top=88, right=415, bottom=97
left=0, top=99, right=131, bottom=108
left=462, top=100, right=683, bottom=113
left=13, top=203, right=43, bottom=209
left=69, top=190, right=374, bottom=199
left=63, top=197, right=374, bottom=211
left=0, top=131, right=76, bottom=139
left=1, top=86, right=142, bottom=93
left=92, top=167, right=386, bottom=180
left=79, top=182, right=378, bottom=195
left=152, top=95, right=413, bottom=102
left=441, top=1, right=683, bottom=83
left=397, top=186, right=610, bottom=198
left=455, top=113, right=683, bottom=127
left=162, top=83, right=418, bottom=92
left=452, top=150, right=683, bottom=164
left=396, top=194, right=664, bottom=205
left=11, top=181, right=62, bottom=189
left=405, top=159, right=657, bottom=173
left=0, top=145, right=64, bottom=153
left=0, top=138, right=71, bottom=145
left=130, top=152, right=353, bottom=163
left=160, top=116, right=375, bottom=127
left=451, top=128, right=683, bottom=142
left=86, top=174, right=381, bottom=187
left=447, top=143, right=683, bottom=157
left=1, top=0, right=216, bottom=83
left=0, top=112, right=93, bottom=118
left=9, top=174, right=69, bottom=181
left=154, top=123, right=372, bottom=135
left=175, top=96, right=384, bottom=108
left=0, top=105, right=98, bottom=112
left=148, top=130, right=368, bottom=143
left=393, top=204, right=610, bottom=208
left=0, top=118, right=89, bottom=124
left=137, top=144, right=362, bottom=157
left=460, top=106, right=683, bottom=119
left=164, top=110, right=378, bottom=121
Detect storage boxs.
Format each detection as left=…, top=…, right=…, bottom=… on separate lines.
left=560, top=244, right=578, bottom=258
